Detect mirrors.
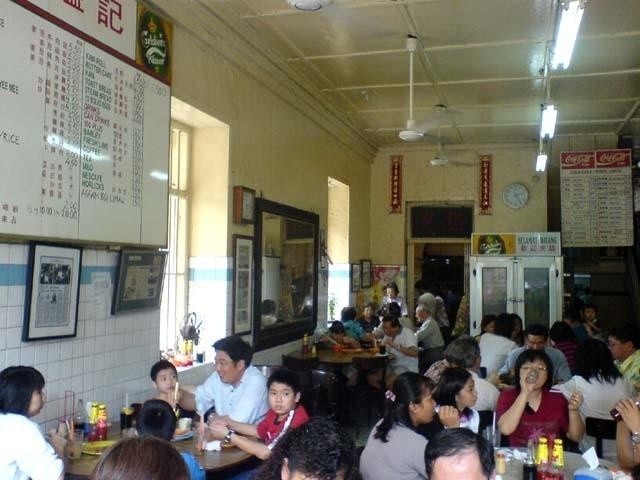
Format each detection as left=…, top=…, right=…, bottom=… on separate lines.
left=250, top=195, right=320, bottom=353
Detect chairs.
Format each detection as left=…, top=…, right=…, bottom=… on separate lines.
left=584, top=418, right=619, bottom=458
left=256, top=353, right=391, bottom=427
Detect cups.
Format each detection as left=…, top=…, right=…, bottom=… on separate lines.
left=379, top=344, right=386, bottom=354
left=118, top=407, right=135, bottom=437
left=483, top=425, right=500, bottom=450
left=191, top=427, right=207, bottom=455
left=66, top=430, right=84, bottom=458
left=526, top=371, right=538, bottom=383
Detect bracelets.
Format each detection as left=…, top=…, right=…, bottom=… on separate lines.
left=208, top=413, right=217, bottom=422
left=223, top=428, right=234, bottom=444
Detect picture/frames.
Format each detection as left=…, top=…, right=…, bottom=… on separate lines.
left=351, top=258, right=372, bottom=293
left=22, top=241, right=83, bottom=343
left=233, top=234, right=252, bottom=335
left=110, top=248, right=170, bottom=314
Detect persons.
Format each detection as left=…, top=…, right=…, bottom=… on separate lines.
left=209, top=366, right=311, bottom=463
left=90, top=432, right=190, bottom=479
left=260, top=297, right=278, bottom=327
left=132, top=398, right=207, bottom=479
left=358, top=303, right=639, bottom=480
left=249, top=416, right=365, bottom=479
left=167, top=333, right=274, bottom=426
left=0, top=364, right=67, bottom=480
left=318, top=277, right=451, bottom=408
left=151, top=360, right=198, bottom=416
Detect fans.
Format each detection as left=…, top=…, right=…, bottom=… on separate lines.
left=283, top=0, right=482, bottom=171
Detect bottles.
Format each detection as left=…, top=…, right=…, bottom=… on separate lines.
left=73, top=399, right=107, bottom=442
left=610, top=399, right=640, bottom=422
left=524, top=439, right=538, bottom=480
left=549, top=439, right=563, bottom=479
left=302, top=334, right=317, bottom=356
left=535, top=438, right=549, bottom=480
left=180, top=339, right=194, bottom=366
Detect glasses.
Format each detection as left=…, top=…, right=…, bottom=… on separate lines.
left=521, top=364, right=547, bottom=372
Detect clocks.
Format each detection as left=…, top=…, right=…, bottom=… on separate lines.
left=502, top=183, right=530, bottom=210
